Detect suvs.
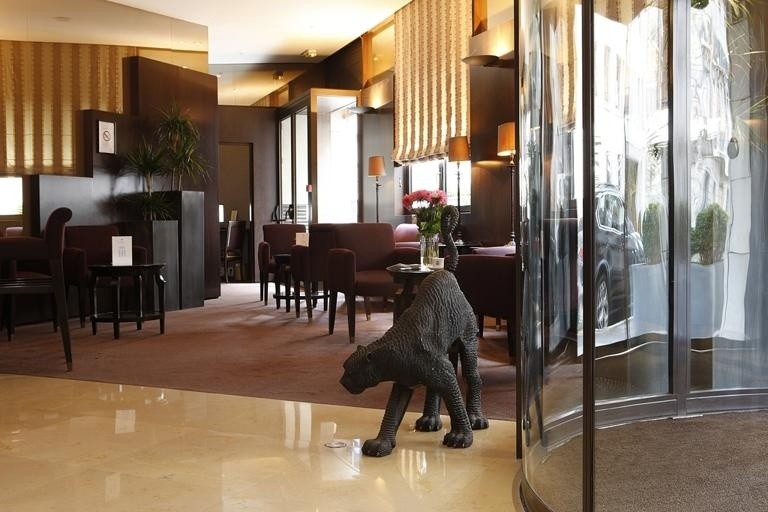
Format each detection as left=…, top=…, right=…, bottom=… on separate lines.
left=557, top=181, right=644, bottom=335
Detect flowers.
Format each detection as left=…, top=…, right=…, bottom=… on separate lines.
left=402, top=190, right=447, bottom=261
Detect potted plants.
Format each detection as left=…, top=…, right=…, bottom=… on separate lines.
left=628, top=203, right=670, bottom=337
left=111, top=134, right=180, bottom=313
left=691, top=205, right=728, bottom=338
left=150, top=96, right=214, bottom=309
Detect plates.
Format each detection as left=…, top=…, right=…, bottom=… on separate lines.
left=428, top=266, right=444, bottom=269
left=398, top=264, right=419, bottom=269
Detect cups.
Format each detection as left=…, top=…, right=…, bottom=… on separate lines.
left=432, top=258, right=444, bottom=266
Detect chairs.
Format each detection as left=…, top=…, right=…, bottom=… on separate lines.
left=0, top=207, right=148, bottom=371
left=221, top=220, right=245, bottom=282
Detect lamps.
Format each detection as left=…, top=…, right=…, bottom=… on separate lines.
left=368, top=156, right=386, bottom=223
left=497, top=122, right=516, bottom=246
left=348, top=107, right=392, bottom=114
left=449, top=136, right=469, bottom=244
left=461, top=55, right=514, bottom=69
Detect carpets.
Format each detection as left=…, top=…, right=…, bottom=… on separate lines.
left=1, top=282, right=517, bottom=422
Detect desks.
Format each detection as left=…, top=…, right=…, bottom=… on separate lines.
left=87, top=264, right=171, bottom=340
